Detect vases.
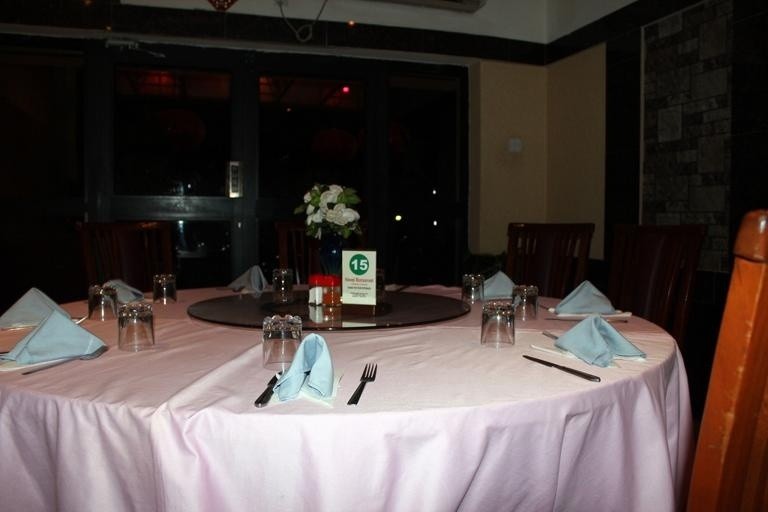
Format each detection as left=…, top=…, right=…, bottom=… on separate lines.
left=315, top=240, right=351, bottom=276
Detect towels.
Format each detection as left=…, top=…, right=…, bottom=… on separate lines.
left=1, top=277, right=144, bottom=369
left=552, top=314, right=647, bottom=369
left=226, top=259, right=268, bottom=297
left=474, top=269, right=517, bottom=302
left=274, top=334, right=339, bottom=406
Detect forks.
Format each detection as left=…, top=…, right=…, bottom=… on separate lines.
left=22, top=346, right=113, bottom=377
left=347, top=363, right=378, bottom=406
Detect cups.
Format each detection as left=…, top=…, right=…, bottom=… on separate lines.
left=87, top=283, right=119, bottom=322
left=479, top=301, right=516, bottom=347
left=117, top=301, right=156, bottom=351
left=463, top=274, right=484, bottom=306
left=512, top=284, right=537, bottom=322
left=272, top=269, right=293, bottom=305
left=263, top=313, right=304, bottom=370
left=153, top=273, right=178, bottom=306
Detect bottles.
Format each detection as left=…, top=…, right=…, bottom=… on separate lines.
left=322, top=275, right=342, bottom=307
left=308, top=276, right=324, bottom=306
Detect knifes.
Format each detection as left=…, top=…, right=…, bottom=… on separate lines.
left=253, top=372, right=282, bottom=408
left=522, top=354, right=601, bottom=384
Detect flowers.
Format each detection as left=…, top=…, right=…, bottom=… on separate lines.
left=294, top=183, right=365, bottom=240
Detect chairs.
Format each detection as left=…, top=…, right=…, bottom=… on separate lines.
left=78, top=219, right=178, bottom=301
left=503, top=220, right=709, bottom=360
left=685, top=208, right=768, bottom=511
left=273, top=217, right=375, bottom=286
